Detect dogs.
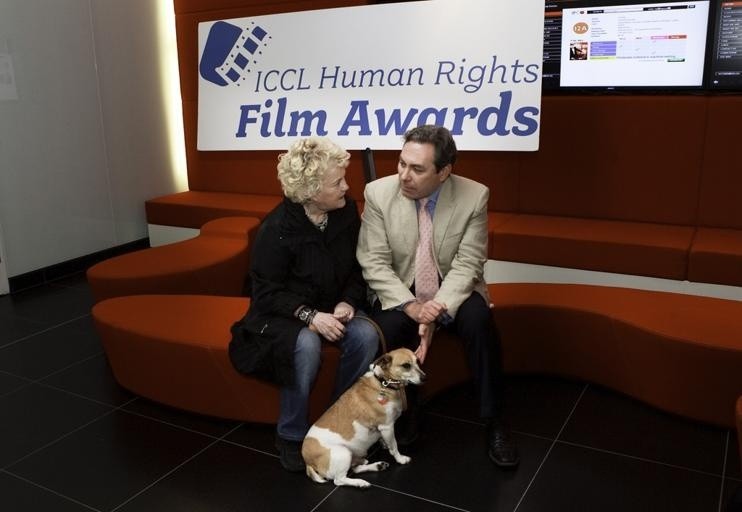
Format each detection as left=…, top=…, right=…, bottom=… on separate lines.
left=300, top=347, right=428, bottom=489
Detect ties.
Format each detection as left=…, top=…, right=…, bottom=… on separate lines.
left=413, top=195, right=439, bottom=303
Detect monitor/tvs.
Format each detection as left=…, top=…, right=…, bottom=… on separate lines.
left=558, top=0, right=713, bottom=92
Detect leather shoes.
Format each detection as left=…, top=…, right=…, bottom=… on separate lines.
left=487, top=422, right=521, bottom=468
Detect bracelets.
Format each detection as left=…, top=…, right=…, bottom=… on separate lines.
left=295, top=305, right=319, bottom=328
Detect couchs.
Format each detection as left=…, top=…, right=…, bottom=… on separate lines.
left=409, top=211, right=741, bottom=428
left=145, top=193, right=284, bottom=247
left=88, top=216, right=261, bottom=302
left=91, top=293, right=340, bottom=424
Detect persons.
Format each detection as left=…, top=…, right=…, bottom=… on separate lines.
left=355, top=124, right=523, bottom=468
left=227, top=137, right=384, bottom=472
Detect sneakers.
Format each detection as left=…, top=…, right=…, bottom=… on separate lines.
left=379, top=427, right=412, bottom=454
left=275, top=435, right=306, bottom=473
